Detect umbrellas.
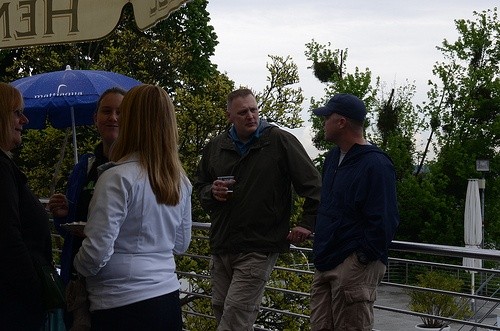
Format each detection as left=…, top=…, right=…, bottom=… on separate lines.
left=462, top=173, right=482, bottom=312
left=8, top=65, right=143, bottom=166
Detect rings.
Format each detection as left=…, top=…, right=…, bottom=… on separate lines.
left=297, top=237, right=301, bottom=239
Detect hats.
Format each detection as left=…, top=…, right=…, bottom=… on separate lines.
left=312, top=94, right=367, bottom=123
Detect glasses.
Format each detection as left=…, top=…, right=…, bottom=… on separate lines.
left=12, top=109, right=24, bottom=116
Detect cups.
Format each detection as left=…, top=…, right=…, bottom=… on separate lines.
left=217, top=176, right=235, bottom=195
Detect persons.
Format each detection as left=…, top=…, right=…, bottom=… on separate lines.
left=309, top=94, right=399, bottom=331
left=48, top=85, right=192, bottom=331
left=195, top=88, right=322, bottom=331
left=0, top=83, right=53, bottom=331
